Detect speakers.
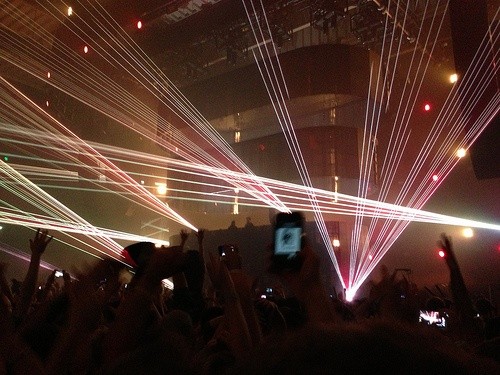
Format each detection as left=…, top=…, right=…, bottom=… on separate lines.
left=449, top=0, right=500, bottom=180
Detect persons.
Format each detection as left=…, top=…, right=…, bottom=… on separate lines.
left=245, top=216, right=254, bottom=227
left=1, top=228, right=499, bottom=375
left=228, top=221, right=237, bottom=229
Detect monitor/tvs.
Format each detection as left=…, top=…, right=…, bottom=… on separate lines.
left=418, top=307, right=448, bottom=329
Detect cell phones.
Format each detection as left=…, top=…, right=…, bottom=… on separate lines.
left=272, top=211, right=305, bottom=274
left=54, top=270, right=64, bottom=277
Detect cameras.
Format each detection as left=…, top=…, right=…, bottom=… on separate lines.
left=260, top=294, right=267, bottom=299
left=265, top=287, right=273, bottom=293
left=217, top=245, right=234, bottom=256
